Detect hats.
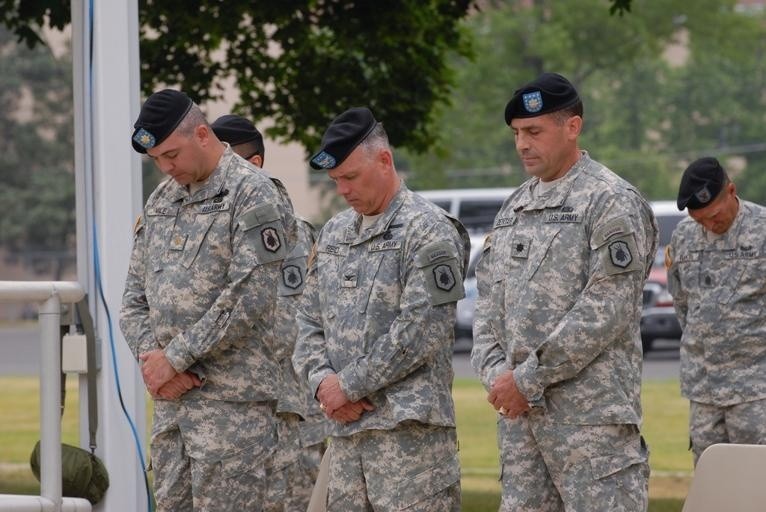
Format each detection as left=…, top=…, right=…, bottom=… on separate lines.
left=504, top=71, right=581, bottom=126
left=210, top=113, right=259, bottom=146
left=131, top=88, right=193, bottom=153
left=676, top=158, right=723, bottom=211
left=309, top=106, right=378, bottom=170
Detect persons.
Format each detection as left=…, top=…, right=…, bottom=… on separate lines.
left=665, top=157, right=766, bottom=469
left=120, top=90, right=297, bottom=512
left=210, top=115, right=316, bottom=512
left=292, top=107, right=471, bottom=512
left=470, top=72, right=659, bottom=512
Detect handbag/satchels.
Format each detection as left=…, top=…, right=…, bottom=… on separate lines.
left=30, top=439, right=110, bottom=506
